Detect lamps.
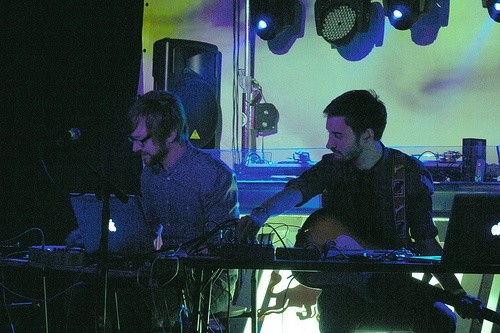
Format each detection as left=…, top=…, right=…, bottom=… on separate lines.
left=383, top=0, right=420, bottom=30
left=251, top=0, right=295, bottom=40
left=314, top=0, right=371, bottom=45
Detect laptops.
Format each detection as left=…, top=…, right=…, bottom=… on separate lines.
left=69, top=193, right=155, bottom=255
left=408, top=193, right=500, bottom=265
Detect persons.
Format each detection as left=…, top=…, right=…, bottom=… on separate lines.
left=236, top=90, right=483, bottom=333
left=62, top=89, right=239, bottom=333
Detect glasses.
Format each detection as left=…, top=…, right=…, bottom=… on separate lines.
left=127, top=134, right=153, bottom=147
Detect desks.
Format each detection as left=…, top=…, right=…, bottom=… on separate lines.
left=148, top=255, right=500, bottom=333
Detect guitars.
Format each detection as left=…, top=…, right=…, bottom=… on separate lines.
left=289, top=206, right=500, bottom=325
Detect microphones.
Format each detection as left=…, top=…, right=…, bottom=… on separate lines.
left=55, top=127, right=81, bottom=144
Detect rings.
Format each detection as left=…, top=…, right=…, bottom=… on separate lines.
left=244, top=219, right=247, bottom=223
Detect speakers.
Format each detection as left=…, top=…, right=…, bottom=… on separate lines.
left=152, top=38, right=222, bottom=158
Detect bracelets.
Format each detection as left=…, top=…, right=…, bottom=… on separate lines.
left=449, top=284, right=462, bottom=295
left=250, top=206, right=269, bottom=219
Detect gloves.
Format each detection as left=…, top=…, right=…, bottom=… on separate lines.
left=451, top=288, right=486, bottom=324
